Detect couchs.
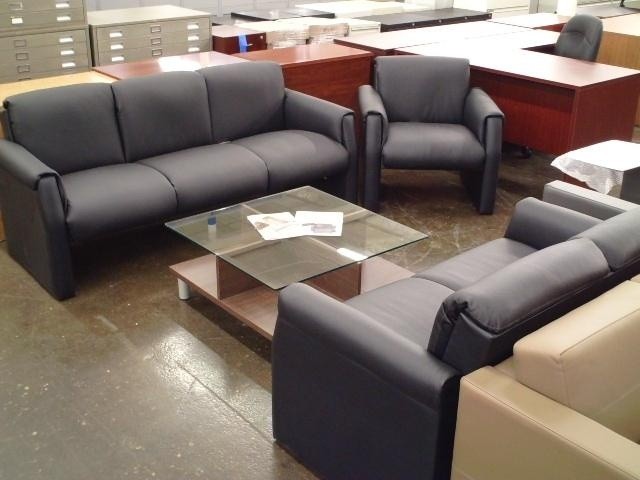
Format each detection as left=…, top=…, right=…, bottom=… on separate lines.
left=275, top=198, right=639, bottom=478
left=444, top=272, right=638, bottom=479
left=0, top=61, right=358, bottom=301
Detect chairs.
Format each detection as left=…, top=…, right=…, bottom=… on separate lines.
left=359, top=53, right=506, bottom=216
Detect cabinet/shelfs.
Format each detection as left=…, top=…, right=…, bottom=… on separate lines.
left=0, top=0, right=211, bottom=84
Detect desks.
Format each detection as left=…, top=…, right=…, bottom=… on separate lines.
left=204, top=1, right=640, bottom=190
left=0, top=71, right=120, bottom=140
